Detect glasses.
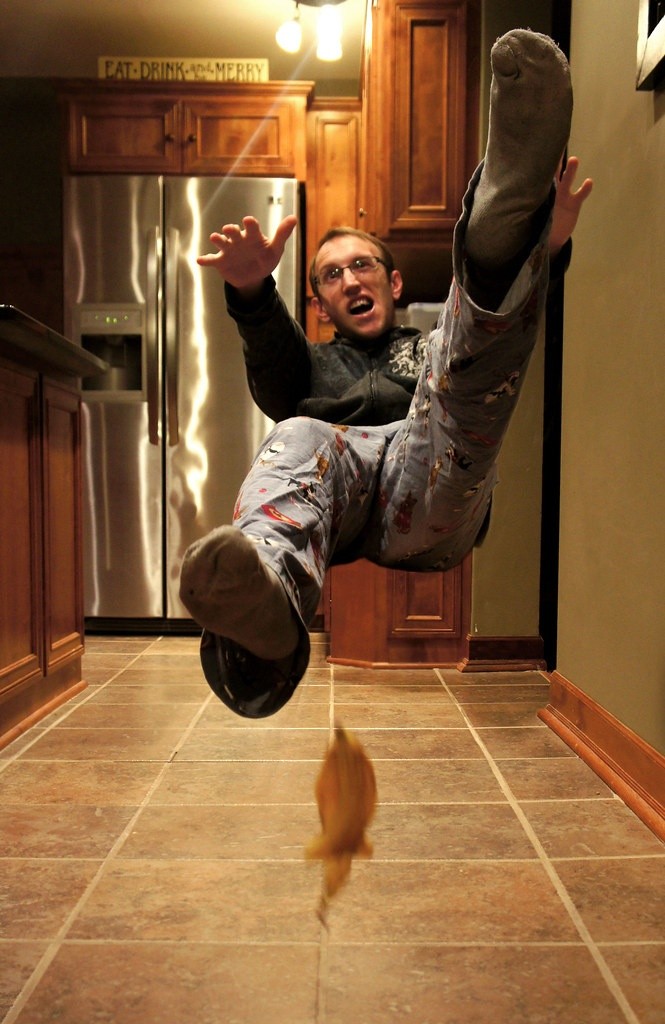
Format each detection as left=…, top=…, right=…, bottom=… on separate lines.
left=312, top=254, right=391, bottom=294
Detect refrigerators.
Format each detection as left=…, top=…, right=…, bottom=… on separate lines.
left=64, top=176, right=300, bottom=638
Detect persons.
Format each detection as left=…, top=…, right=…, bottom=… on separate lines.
left=179, top=30, right=593, bottom=719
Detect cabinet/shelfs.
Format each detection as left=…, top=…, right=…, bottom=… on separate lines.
left=1, top=341, right=88, bottom=746
left=64, top=0, right=482, bottom=672
